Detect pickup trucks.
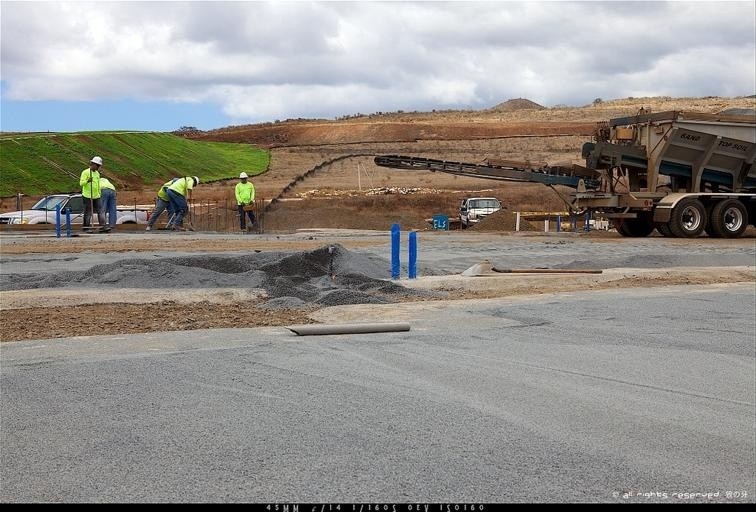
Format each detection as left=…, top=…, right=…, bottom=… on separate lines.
left=0, top=194, right=151, bottom=231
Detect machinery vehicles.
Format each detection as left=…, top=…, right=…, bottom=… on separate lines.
left=575, top=106, right=754, bottom=238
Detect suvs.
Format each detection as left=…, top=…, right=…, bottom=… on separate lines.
left=458, top=194, right=502, bottom=229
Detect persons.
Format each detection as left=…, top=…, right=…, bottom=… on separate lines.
left=146, top=178, right=180, bottom=231
left=235, top=172, right=257, bottom=232
left=98, top=177, right=116, bottom=228
left=167, top=175, right=199, bottom=231
left=79, top=156, right=111, bottom=233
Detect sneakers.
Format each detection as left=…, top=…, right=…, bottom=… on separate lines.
left=84, top=228, right=94, bottom=234
left=171, top=223, right=185, bottom=231
left=144, top=225, right=152, bottom=231
left=99, top=226, right=112, bottom=233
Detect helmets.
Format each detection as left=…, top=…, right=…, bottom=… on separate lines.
left=239, top=172, right=248, bottom=179
left=89, top=156, right=103, bottom=167
left=190, top=175, right=200, bottom=185
